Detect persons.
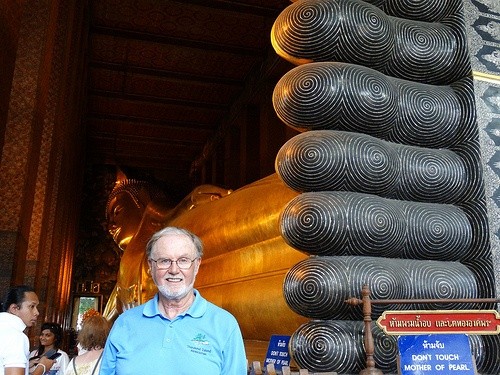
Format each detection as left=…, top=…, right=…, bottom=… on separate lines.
left=30, top=322, right=70, bottom=375
left=0, top=286, right=56, bottom=375
left=66, top=316, right=112, bottom=375
left=99, top=227, right=247, bottom=375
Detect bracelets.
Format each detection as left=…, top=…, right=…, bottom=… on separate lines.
left=34, top=363, right=46, bottom=375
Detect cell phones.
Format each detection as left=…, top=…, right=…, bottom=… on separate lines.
left=48, top=352, right=62, bottom=360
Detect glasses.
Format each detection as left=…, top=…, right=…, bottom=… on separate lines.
left=148, top=256, right=198, bottom=270
